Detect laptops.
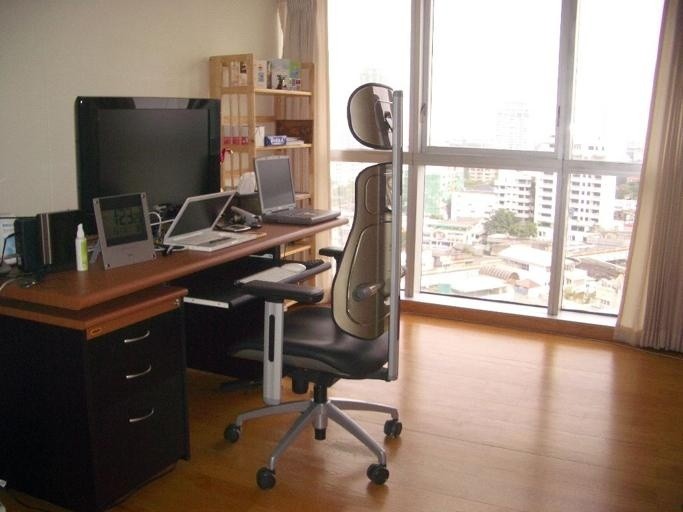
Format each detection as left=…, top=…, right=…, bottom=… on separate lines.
left=163, top=190, right=267, bottom=252
left=254, top=155, right=341, bottom=224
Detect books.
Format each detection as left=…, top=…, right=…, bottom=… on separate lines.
left=286, top=136, right=306, bottom=147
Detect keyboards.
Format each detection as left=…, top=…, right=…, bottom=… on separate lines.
left=239, top=267, right=300, bottom=288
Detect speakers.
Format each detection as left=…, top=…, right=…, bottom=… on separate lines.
left=14, top=211, right=87, bottom=273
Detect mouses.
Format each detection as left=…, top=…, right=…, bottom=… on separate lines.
left=282, top=263, right=306, bottom=272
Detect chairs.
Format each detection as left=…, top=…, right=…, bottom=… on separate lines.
left=225, top=84, right=404, bottom=491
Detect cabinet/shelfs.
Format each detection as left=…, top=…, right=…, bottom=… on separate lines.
left=209, top=49, right=319, bottom=298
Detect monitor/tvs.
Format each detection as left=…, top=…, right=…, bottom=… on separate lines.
left=75, top=96, right=221, bottom=236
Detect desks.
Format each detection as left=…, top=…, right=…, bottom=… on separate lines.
left=0, top=202, right=348, bottom=512
